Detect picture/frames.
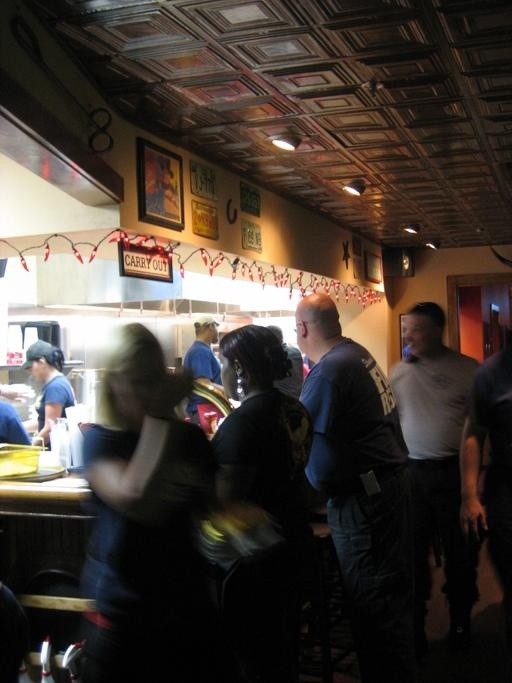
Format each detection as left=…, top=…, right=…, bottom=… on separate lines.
left=364, top=249, right=383, bottom=283
left=135, top=136, right=186, bottom=232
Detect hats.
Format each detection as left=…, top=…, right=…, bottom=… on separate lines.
left=21, top=340, right=53, bottom=370
left=193, top=315, right=219, bottom=330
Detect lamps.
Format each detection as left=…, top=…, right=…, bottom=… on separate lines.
left=344, top=179, right=366, bottom=197
left=403, top=222, right=442, bottom=250
left=270, top=135, right=301, bottom=151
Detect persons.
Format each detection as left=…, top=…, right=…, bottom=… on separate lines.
left=0, top=341, right=74, bottom=444
left=77, top=291, right=512, bottom=682
